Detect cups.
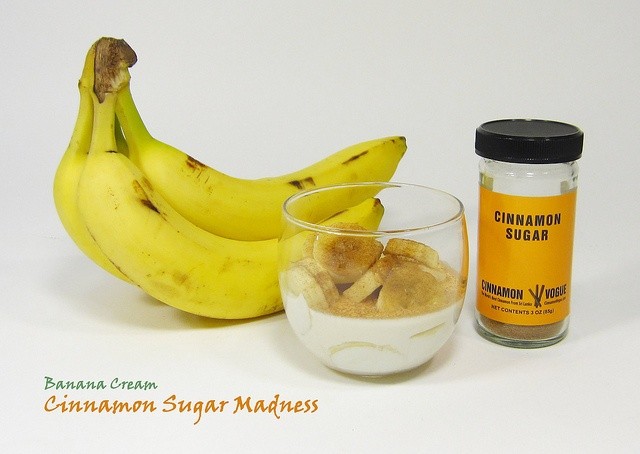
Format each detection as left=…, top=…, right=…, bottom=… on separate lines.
left=278, top=181, right=469, bottom=375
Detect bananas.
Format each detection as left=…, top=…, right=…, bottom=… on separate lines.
left=52, top=37, right=408, bottom=320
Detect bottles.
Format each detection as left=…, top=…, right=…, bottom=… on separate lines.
left=474, top=119, right=584, bottom=349
